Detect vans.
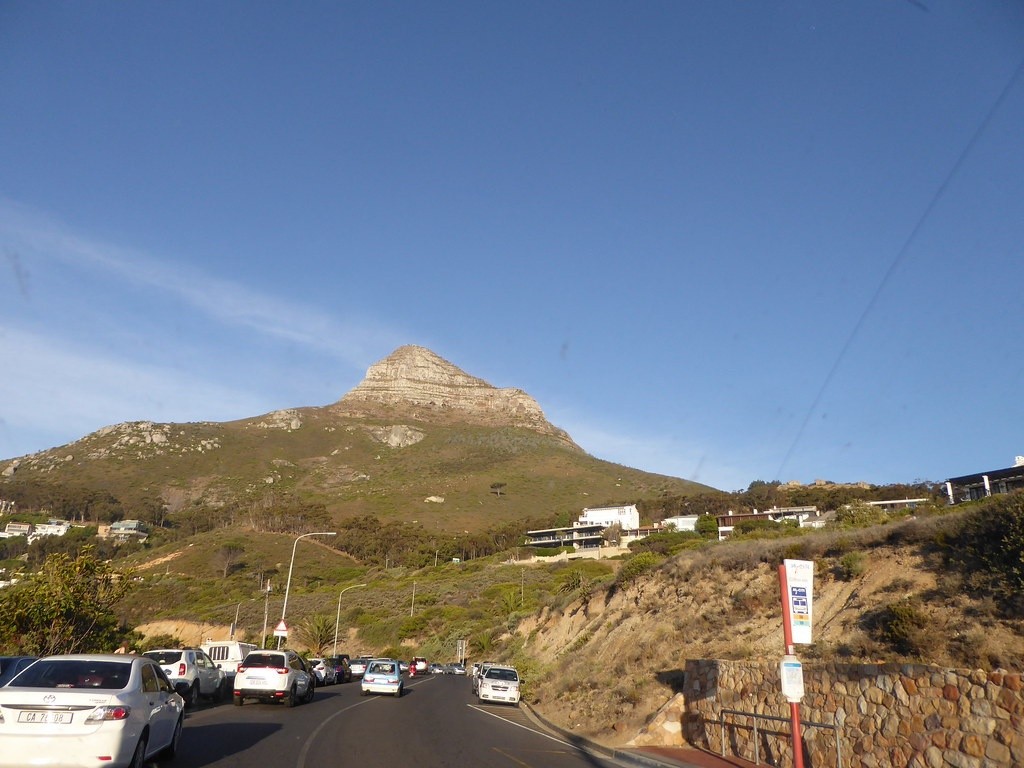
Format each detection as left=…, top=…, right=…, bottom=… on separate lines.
left=193, top=640, right=259, bottom=689
left=414, top=656, right=428, bottom=675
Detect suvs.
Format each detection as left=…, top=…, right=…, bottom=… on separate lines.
left=141, top=646, right=229, bottom=709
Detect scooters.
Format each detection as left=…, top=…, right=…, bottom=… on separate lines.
left=409, top=670, right=416, bottom=680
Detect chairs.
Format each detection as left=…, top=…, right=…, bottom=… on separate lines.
left=374, top=667, right=380, bottom=673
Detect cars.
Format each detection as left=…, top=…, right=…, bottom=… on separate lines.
left=360, top=660, right=405, bottom=698
left=304, top=654, right=410, bottom=687
left=472, top=661, right=525, bottom=707
left=429, top=662, right=444, bottom=674
left=233, top=649, right=316, bottom=708
left=0, top=653, right=187, bottom=768
left=444, top=662, right=467, bottom=675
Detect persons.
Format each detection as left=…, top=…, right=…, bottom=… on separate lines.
left=408, top=656, right=418, bottom=666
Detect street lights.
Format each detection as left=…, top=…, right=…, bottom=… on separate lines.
left=277, top=531, right=336, bottom=651
left=332, top=583, right=366, bottom=658
